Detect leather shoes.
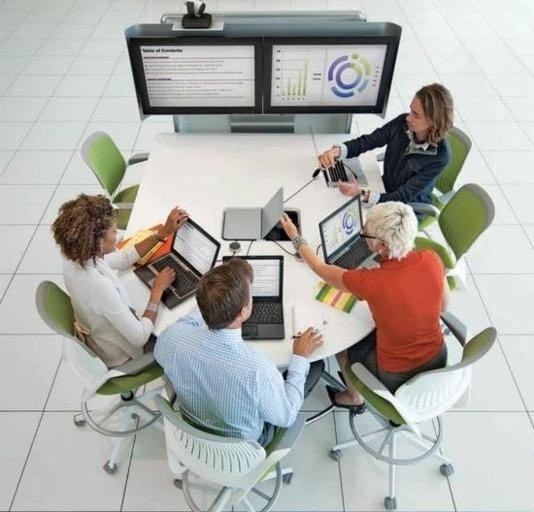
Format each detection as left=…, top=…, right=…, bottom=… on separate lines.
left=324, top=384, right=366, bottom=415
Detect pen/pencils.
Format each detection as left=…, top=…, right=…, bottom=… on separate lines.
left=292, top=335, right=315, bottom=338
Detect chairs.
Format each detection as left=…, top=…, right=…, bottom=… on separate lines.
left=80, top=132, right=151, bottom=230
left=406, top=182, right=495, bottom=291
left=329, top=310, right=497, bottom=509
left=36, top=281, right=165, bottom=475
left=152, top=394, right=294, bottom=512
left=376, top=126, right=472, bottom=241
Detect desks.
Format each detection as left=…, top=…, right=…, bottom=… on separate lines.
left=117, top=132, right=385, bottom=401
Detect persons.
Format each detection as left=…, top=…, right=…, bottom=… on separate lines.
left=151, top=259, right=326, bottom=453
left=277, top=198, right=448, bottom=418
left=315, top=82, right=455, bottom=220
left=51, top=193, right=190, bottom=373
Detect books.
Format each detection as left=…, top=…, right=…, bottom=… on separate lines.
left=291, top=301, right=324, bottom=339
left=113, top=222, right=174, bottom=271
left=314, top=278, right=359, bottom=314
left=120, top=228, right=168, bottom=264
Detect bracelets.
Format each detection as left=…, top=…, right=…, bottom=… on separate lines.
left=154, top=231, right=165, bottom=243
left=360, top=189, right=368, bottom=201
left=290, top=235, right=306, bottom=251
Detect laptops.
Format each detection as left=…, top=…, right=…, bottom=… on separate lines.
left=319, top=194, right=375, bottom=269
left=309, top=124, right=368, bottom=188
left=133, top=216, right=221, bottom=310
left=223, top=255, right=285, bottom=340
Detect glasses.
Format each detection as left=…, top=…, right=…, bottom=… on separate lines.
left=359, top=227, right=378, bottom=240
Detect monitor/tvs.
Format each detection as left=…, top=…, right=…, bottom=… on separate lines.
left=264, top=36, right=395, bottom=114
left=128, top=38, right=264, bottom=114
